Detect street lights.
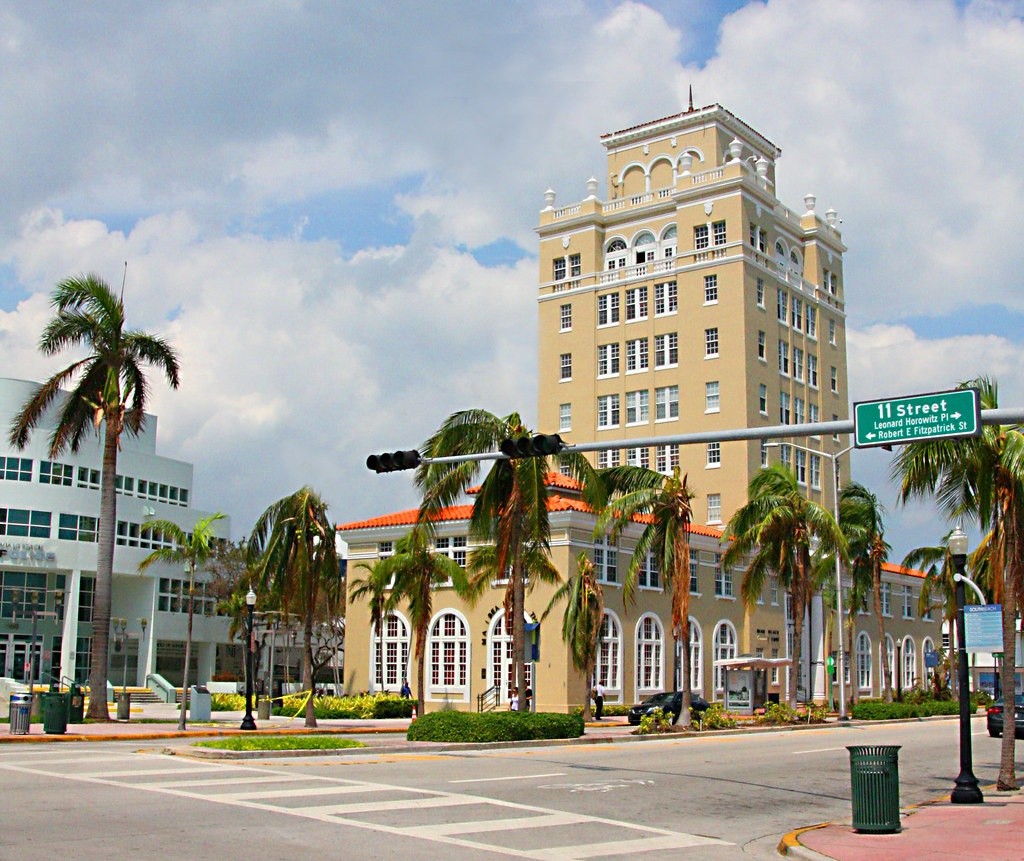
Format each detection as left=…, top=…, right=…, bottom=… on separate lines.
left=896, top=639, right=903, bottom=702
left=240, top=589, right=257, bottom=730
left=948, top=523, right=985, bottom=804
left=763, top=442, right=892, bottom=721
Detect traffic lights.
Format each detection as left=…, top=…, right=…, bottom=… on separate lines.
left=366, top=449, right=421, bottom=473
left=501, top=434, right=564, bottom=459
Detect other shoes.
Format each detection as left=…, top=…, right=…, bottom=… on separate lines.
left=596, top=717, right=602, bottom=720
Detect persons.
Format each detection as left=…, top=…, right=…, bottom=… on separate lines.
left=507, top=681, right=533, bottom=711
left=593, top=681, right=607, bottom=720
left=401, top=682, right=414, bottom=699
left=728, top=687, right=749, bottom=705
left=981, top=682, right=995, bottom=695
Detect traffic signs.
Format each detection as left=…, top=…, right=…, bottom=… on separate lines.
left=853, top=386, right=983, bottom=450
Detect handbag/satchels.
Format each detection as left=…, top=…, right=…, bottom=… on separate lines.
left=591, top=686, right=597, bottom=700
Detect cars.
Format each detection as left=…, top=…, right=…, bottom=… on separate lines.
left=628, top=692, right=710, bottom=726
left=987, top=694, right=1024, bottom=737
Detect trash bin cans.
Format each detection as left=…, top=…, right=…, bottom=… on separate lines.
left=43, top=691, right=68, bottom=733
left=40, top=681, right=85, bottom=724
left=189, top=685, right=211, bottom=721
left=845, top=745, right=904, bottom=833
left=258, top=695, right=269, bottom=719
left=116, top=692, right=131, bottom=719
left=9, top=693, right=33, bottom=733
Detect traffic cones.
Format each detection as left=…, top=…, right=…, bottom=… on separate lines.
left=411, top=706, right=417, bottom=722
